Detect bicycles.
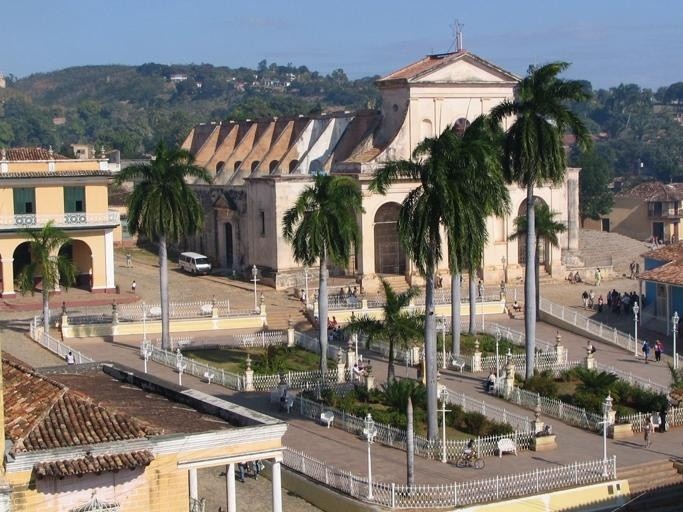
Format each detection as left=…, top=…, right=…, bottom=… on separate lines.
left=455, top=451, right=487, bottom=470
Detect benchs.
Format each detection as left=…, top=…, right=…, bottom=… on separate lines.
left=327, top=293, right=357, bottom=303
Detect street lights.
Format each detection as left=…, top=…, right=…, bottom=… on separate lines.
left=479, top=282, right=487, bottom=331
left=438, top=384, right=452, bottom=464
left=631, top=301, right=640, bottom=357
left=302, top=263, right=312, bottom=309
left=175, top=348, right=184, bottom=386
left=440, top=313, right=449, bottom=370
left=495, top=325, right=503, bottom=378
left=362, top=413, right=378, bottom=501
left=670, top=310, right=681, bottom=370
left=138, top=300, right=151, bottom=374
left=249, top=264, right=261, bottom=313
left=599, top=402, right=612, bottom=478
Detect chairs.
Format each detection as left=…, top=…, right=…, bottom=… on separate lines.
left=487, top=371, right=499, bottom=390
left=354, top=369, right=368, bottom=381
left=201, top=304, right=213, bottom=314
left=320, top=410, right=335, bottom=429
left=450, top=356, right=466, bottom=374
left=280, top=396, right=294, bottom=414
left=149, top=306, right=162, bottom=319
left=496, top=438, right=517, bottom=458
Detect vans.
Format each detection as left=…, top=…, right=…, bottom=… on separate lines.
left=179, top=251, right=212, bottom=276
